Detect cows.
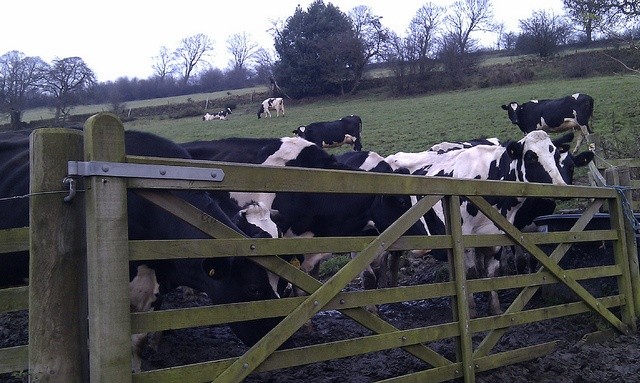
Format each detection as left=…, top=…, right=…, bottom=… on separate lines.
left=427, top=137, right=593, bottom=232
left=292, top=115, right=364, bottom=152
left=351, top=130, right=595, bottom=318
left=502, top=93, right=595, bottom=154
left=202, top=107, right=232, bottom=121
left=173, top=136, right=396, bottom=334
left=257, top=97, right=286, bottom=120
left=0, top=129, right=286, bottom=371
